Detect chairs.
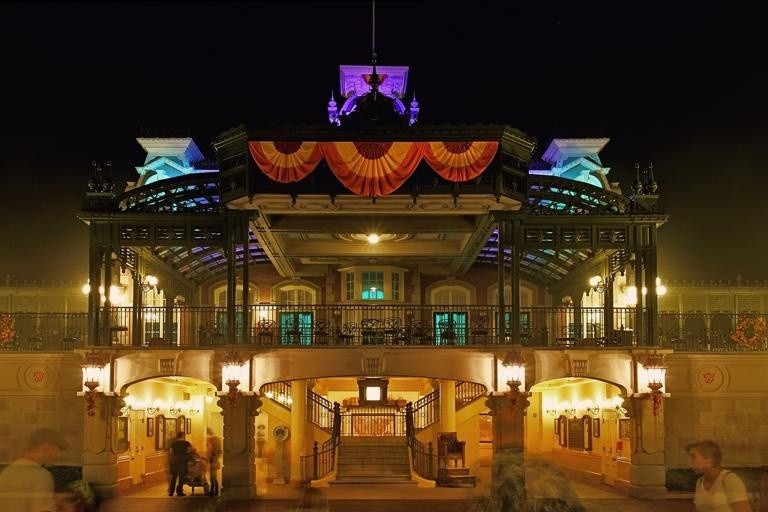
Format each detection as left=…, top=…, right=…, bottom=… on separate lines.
left=437, top=432, right=465, bottom=467
left=205, top=320, right=226, bottom=345
left=258, top=315, right=625, bottom=345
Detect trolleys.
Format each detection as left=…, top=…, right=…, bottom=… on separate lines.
left=182, top=449, right=210, bottom=494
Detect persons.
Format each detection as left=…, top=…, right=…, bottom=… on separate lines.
left=681, top=440, right=754, bottom=512
left=1, top=429, right=68, bottom=511
left=165, top=427, right=221, bottom=499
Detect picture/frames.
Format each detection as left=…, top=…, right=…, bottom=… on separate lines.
left=554, top=415, right=600, bottom=451
left=147, top=414, right=191, bottom=451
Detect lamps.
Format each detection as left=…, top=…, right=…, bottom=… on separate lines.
left=122, top=396, right=200, bottom=415
left=590, top=276, right=607, bottom=293
left=80, top=344, right=106, bottom=416
left=547, top=398, right=625, bottom=415
left=501, top=347, right=527, bottom=416
left=220, top=347, right=245, bottom=408
left=139, top=274, right=158, bottom=293
left=643, top=350, right=668, bottom=416
